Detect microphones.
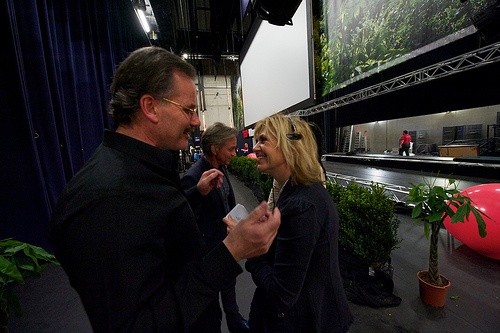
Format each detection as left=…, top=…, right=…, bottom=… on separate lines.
left=259, top=171, right=267, bottom=182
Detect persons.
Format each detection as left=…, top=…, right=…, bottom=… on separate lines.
left=221, top=113, right=354, bottom=333
left=178, top=121, right=252, bottom=333
left=399, top=130, right=412, bottom=156
left=49, top=46, right=281, bottom=333
left=179, top=141, right=256, bottom=170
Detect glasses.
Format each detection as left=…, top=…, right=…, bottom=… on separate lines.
left=161, top=97, right=197, bottom=120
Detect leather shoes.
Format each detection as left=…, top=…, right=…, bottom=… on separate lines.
left=228, top=318, right=249, bottom=333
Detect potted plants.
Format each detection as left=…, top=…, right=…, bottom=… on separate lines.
left=399, top=170, right=496, bottom=309
